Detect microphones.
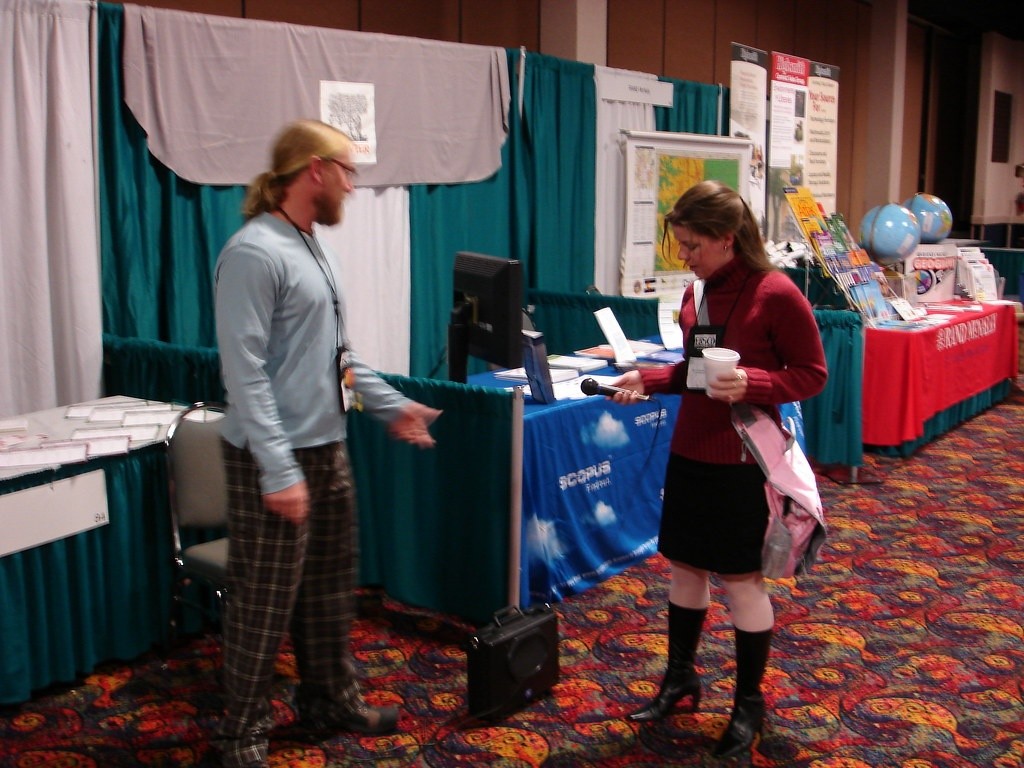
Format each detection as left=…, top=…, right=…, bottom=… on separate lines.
left=581, top=378, right=658, bottom=402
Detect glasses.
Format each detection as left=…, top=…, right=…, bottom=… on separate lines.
left=323, top=157, right=359, bottom=179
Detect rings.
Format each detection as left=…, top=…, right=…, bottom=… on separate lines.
left=735, top=371, right=742, bottom=380
left=728, top=396, right=733, bottom=404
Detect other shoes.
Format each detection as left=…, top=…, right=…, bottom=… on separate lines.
left=337, top=702, right=397, bottom=733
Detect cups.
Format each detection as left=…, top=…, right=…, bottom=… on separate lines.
left=702, top=347, right=741, bottom=399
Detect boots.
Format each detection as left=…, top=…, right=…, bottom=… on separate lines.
left=627, top=600, right=708, bottom=721
left=713, top=624, right=773, bottom=758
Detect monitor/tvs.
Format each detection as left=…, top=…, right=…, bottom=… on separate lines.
left=449, top=253, right=524, bottom=382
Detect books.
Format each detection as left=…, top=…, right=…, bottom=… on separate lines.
left=783, top=185, right=997, bottom=320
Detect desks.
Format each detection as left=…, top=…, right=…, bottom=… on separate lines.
left=861, top=297, right=1020, bottom=458
left=463, top=335, right=804, bottom=610
left=0, top=396, right=230, bottom=705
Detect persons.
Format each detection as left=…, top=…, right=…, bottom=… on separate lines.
left=605, top=179, right=827, bottom=758
left=209, top=116, right=442, bottom=768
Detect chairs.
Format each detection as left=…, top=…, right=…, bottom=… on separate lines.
left=166, top=402, right=231, bottom=659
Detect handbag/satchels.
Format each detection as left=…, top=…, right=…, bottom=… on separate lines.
left=732, top=412, right=827, bottom=581
left=465, top=604, right=562, bottom=724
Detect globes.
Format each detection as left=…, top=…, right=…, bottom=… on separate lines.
left=903, top=192, right=953, bottom=244
left=859, top=203, right=921, bottom=276
